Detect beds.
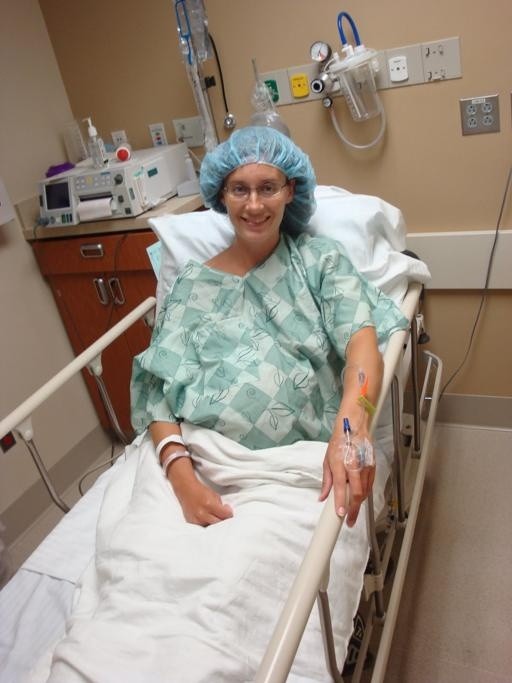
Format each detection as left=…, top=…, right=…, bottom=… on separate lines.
left=2, top=277, right=442, bottom=677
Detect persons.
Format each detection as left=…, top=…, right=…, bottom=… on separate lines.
left=130, top=124, right=385, bottom=531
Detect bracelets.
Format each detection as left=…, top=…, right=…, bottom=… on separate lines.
left=161, top=450, right=191, bottom=478
left=154, top=433, right=187, bottom=466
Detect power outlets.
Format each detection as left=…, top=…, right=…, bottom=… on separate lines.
left=459, top=92, right=500, bottom=135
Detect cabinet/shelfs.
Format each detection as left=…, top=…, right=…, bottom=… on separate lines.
left=29, top=228, right=158, bottom=443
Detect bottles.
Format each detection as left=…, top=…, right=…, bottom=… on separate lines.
left=116, top=141, right=132, bottom=160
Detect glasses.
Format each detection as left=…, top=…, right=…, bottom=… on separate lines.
left=224, top=181, right=289, bottom=201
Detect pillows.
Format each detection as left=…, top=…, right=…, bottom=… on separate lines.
left=149, top=184, right=432, bottom=348
